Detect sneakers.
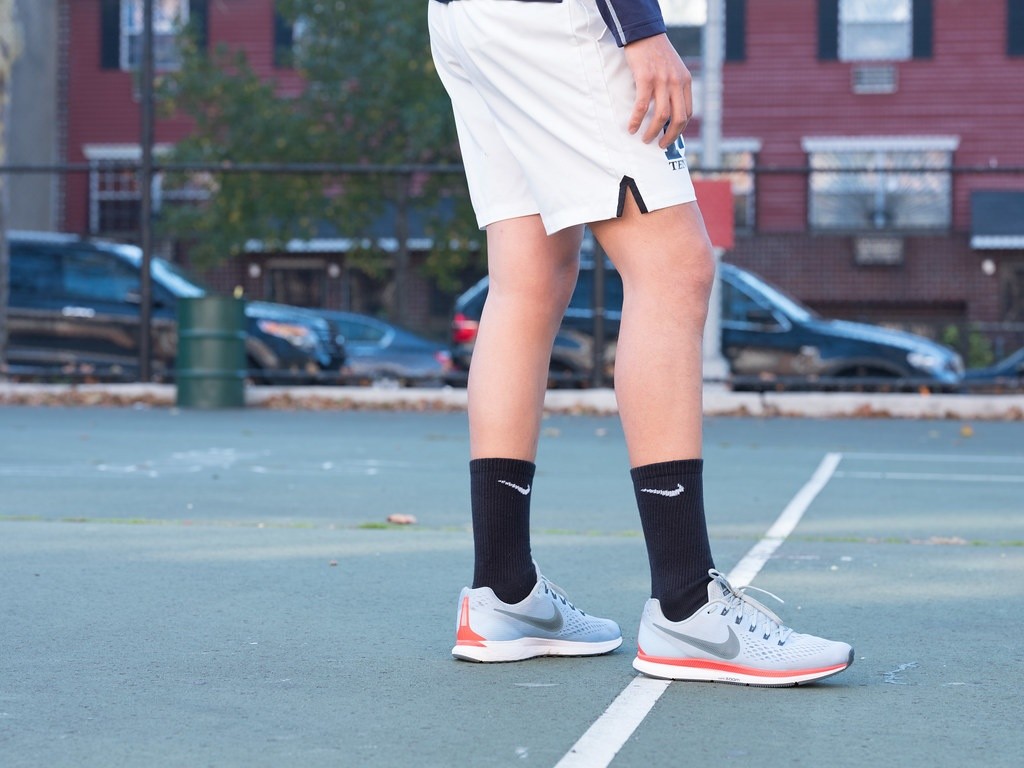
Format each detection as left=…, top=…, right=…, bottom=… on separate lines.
left=633, top=566, right=854, bottom=687
left=452, top=558, right=623, bottom=662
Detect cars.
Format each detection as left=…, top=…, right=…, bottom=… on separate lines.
left=964, top=346, right=1024, bottom=393
left=320, top=308, right=455, bottom=386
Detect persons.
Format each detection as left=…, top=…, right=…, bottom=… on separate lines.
left=426, top=0, right=858, bottom=687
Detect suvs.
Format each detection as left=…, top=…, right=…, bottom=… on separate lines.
left=8, top=229, right=345, bottom=388
left=451, top=242, right=966, bottom=395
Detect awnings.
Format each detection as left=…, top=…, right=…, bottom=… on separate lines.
left=240, top=194, right=597, bottom=254
left=969, top=191, right=1024, bottom=251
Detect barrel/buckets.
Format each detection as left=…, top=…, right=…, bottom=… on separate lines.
left=176, top=296, right=250, bottom=407
